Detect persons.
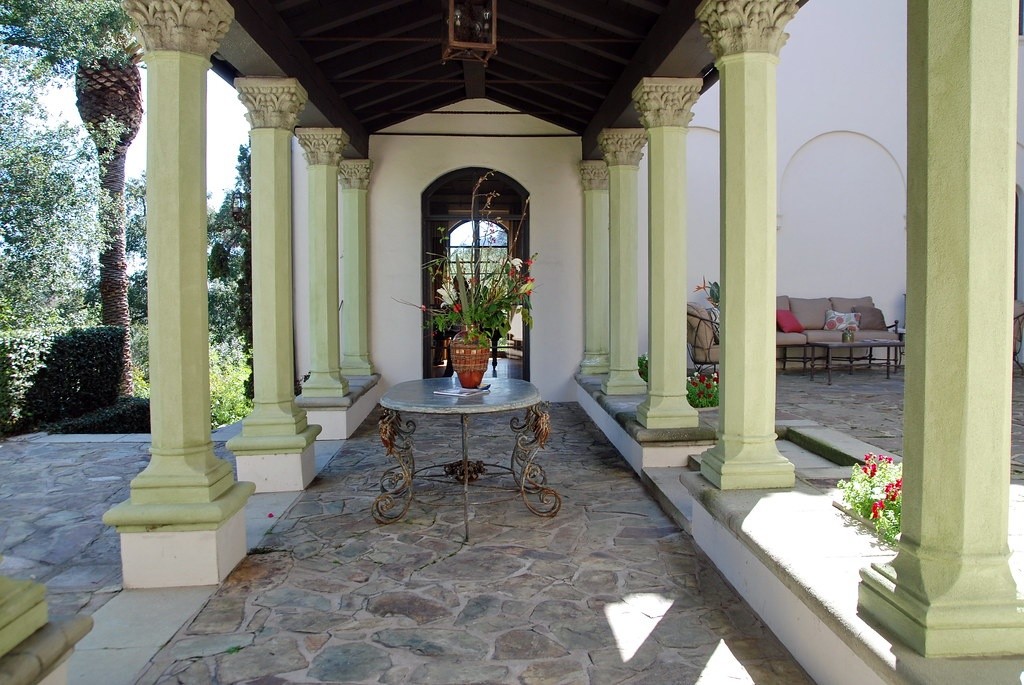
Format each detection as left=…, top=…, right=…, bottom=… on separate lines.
left=433, top=323, right=444, bottom=366
left=443, top=276, right=475, bottom=378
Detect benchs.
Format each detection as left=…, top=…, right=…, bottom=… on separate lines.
left=776, top=295, right=899, bottom=376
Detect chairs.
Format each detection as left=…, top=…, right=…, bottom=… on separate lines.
left=687, top=310, right=720, bottom=374
left=1013, top=312, right=1024, bottom=376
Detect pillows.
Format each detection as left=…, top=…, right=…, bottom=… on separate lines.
left=776, top=308, right=804, bottom=333
left=823, top=309, right=863, bottom=331
left=851, top=306, right=888, bottom=331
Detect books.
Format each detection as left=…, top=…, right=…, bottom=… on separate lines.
left=434, top=384, right=492, bottom=397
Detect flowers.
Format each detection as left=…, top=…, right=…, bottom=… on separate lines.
left=638, top=351, right=648, bottom=382
left=836, top=323, right=858, bottom=335
left=686, top=371, right=719, bottom=408
left=390, top=169, right=545, bottom=350
left=837, top=451, right=903, bottom=546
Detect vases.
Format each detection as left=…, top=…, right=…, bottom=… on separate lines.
left=450, top=323, right=491, bottom=387
left=841, top=330, right=855, bottom=343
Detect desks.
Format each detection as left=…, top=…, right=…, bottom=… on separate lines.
left=806, top=338, right=905, bottom=385
left=371, top=377, right=562, bottom=543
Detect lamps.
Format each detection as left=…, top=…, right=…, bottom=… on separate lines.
left=441, top=0, right=498, bottom=69
